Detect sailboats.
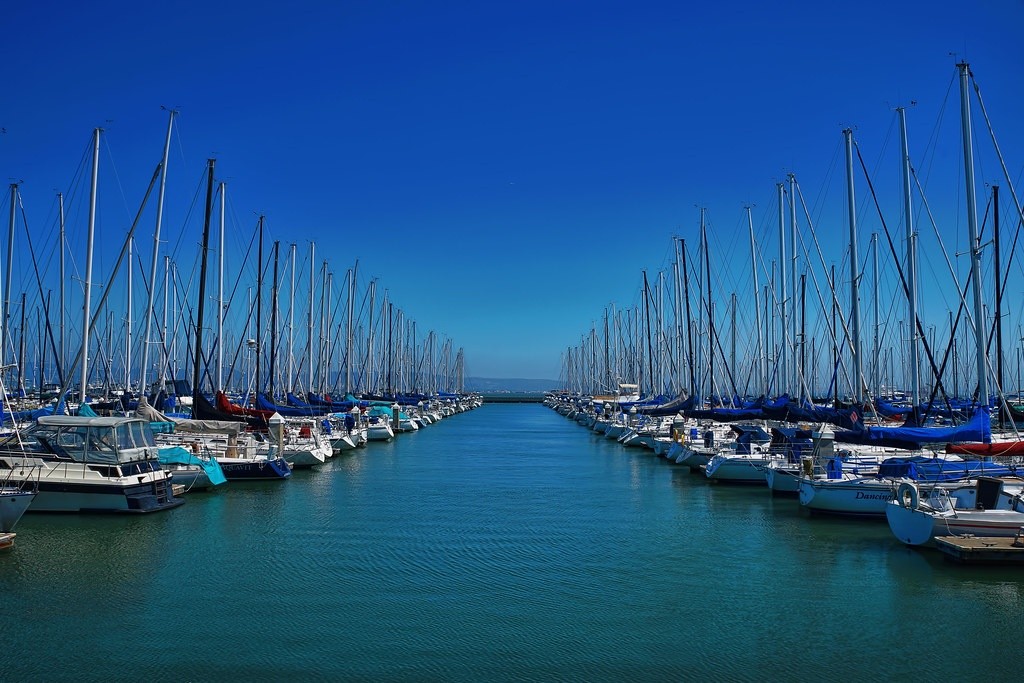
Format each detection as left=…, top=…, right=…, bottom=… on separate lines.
left=542, top=61, right=1024, bottom=562
left=0, top=106, right=485, bottom=551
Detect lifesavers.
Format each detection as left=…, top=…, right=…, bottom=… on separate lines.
left=674, top=430, right=679, bottom=442
left=681, top=434, right=684, bottom=445
left=898, top=482, right=921, bottom=510
